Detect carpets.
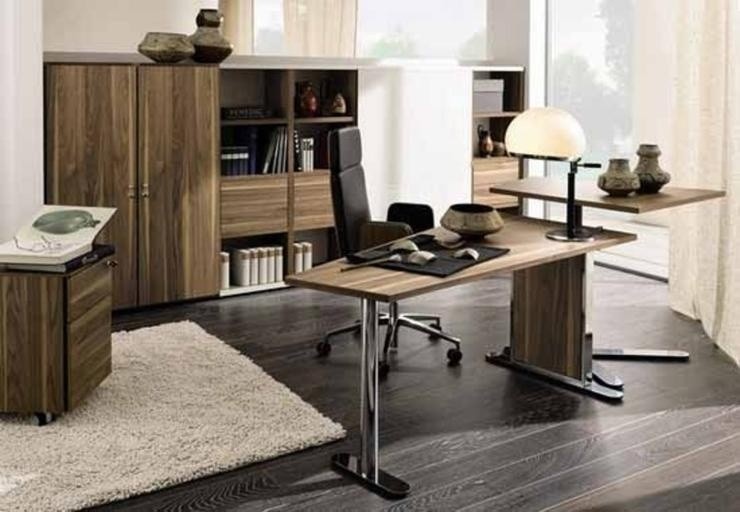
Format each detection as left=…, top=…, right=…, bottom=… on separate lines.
left=1, top=318, right=349, bottom=512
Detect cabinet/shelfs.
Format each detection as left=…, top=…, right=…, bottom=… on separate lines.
left=44, top=50, right=526, bottom=312
left=1, top=244, right=119, bottom=427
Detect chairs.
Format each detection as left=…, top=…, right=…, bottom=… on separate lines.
left=316, top=125, right=463, bottom=376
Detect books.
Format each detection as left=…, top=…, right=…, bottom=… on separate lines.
left=257, top=246, right=267, bottom=285
left=266, top=247, right=276, bottom=283
left=300, top=241, right=313, bottom=272
left=293, top=242, right=303, bottom=273
left=274, top=246, right=284, bottom=282
left=231, top=247, right=250, bottom=286
left=220, top=251, right=230, bottom=289
left=0, top=203, right=121, bottom=266
left=220, top=129, right=314, bottom=177
left=221, top=107, right=277, bottom=120
left=246, top=247, right=259, bottom=285
left=6, top=244, right=116, bottom=274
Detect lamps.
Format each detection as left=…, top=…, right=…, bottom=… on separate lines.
left=505, top=104, right=603, bottom=243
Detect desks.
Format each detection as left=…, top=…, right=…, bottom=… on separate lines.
left=284, top=212, right=638, bottom=499
left=489, top=176, right=727, bottom=389
